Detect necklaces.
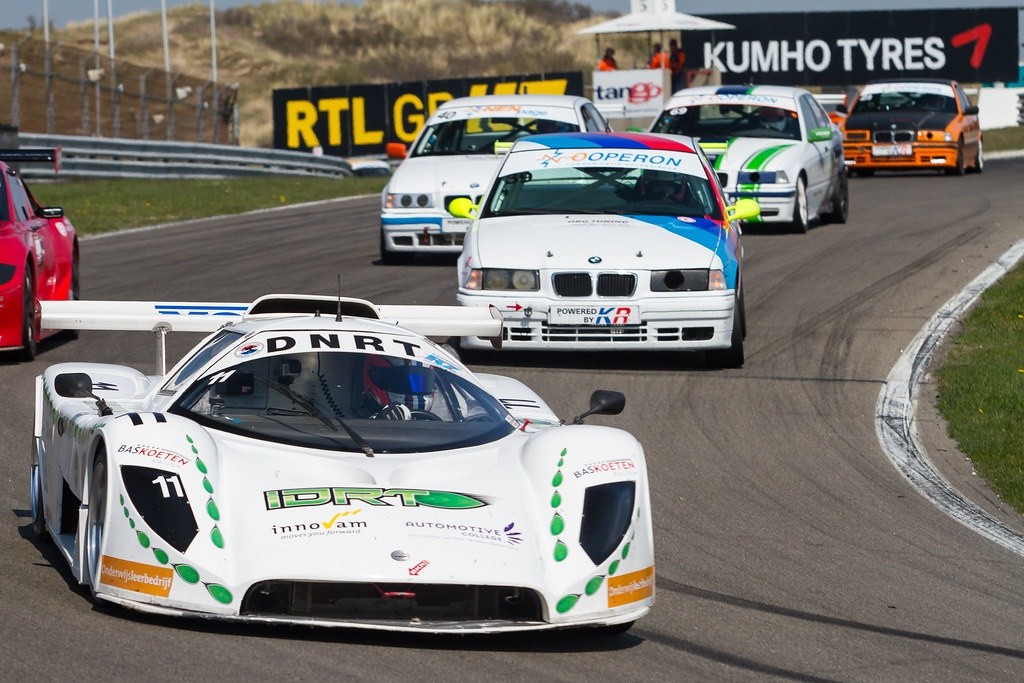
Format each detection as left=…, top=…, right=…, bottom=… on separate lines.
left=641, top=169, right=686, bottom=204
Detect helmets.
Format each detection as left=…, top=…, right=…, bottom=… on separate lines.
left=918, top=94, right=948, bottom=110
left=756, top=108, right=787, bottom=132
left=361, top=353, right=439, bottom=412
left=640, top=169, right=687, bottom=202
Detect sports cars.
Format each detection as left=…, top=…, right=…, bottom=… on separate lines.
left=27, top=291, right=658, bottom=636
left=378, top=93, right=611, bottom=266
left=1, top=144, right=81, bottom=362
left=841, top=77, right=985, bottom=177
left=450, top=132, right=759, bottom=371
left=809, top=92, right=849, bottom=127
left=628, top=85, right=850, bottom=234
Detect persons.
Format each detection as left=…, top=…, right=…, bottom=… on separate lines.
left=648, top=40, right=688, bottom=95
left=599, top=48, right=617, bottom=70
left=759, top=106, right=787, bottom=131
left=363, top=352, right=436, bottom=420
left=923, top=94, right=947, bottom=111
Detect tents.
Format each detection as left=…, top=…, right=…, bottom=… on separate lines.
left=576, top=10, right=736, bottom=120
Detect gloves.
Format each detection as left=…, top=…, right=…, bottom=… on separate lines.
left=371, top=403, right=412, bottom=420
